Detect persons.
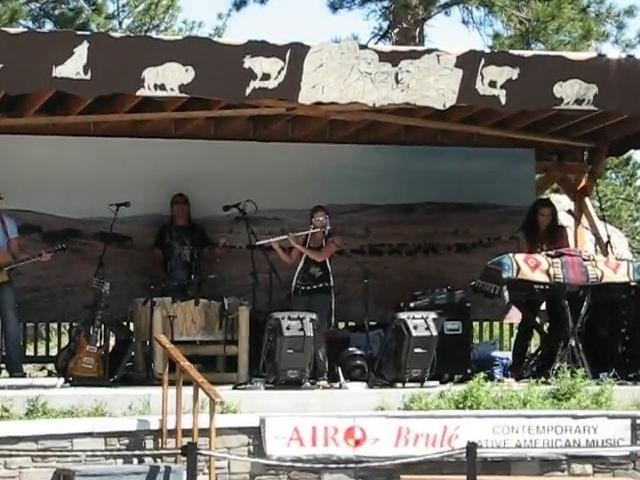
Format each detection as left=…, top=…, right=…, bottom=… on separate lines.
left=154, top=191, right=225, bottom=297
left=508, top=198, right=571, bottom=382
left=0, top=196, right=53, bottom=378
left=271, top=205, right=344, bottom=387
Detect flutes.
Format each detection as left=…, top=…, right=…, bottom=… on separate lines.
left=256, top=226, right=331, bottom=245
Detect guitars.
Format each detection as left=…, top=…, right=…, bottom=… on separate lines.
left=55, top=277, right=112, bottom=386
left=0, top=239, right=69, bottom=283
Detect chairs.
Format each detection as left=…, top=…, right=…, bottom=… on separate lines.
left=132, top=297, right=250, bottom=385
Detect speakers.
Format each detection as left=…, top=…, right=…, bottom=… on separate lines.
left=368, top=312, right=437, bottom=389
left=262, top=311, right=316, bottom=386
left=416, top=303, right=473, bottom=380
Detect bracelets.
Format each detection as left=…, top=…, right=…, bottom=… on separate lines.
left=273, top=246, right=281, bottom=252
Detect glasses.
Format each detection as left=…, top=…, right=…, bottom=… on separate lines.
left=313, top=215, right=329, bottom=224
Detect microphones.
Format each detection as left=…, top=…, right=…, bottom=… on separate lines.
left=222, top=201, right=244, bottom=213
left=112, top=201, right=130, bottom=208
left=566, top=209, right=577, bottom=220
